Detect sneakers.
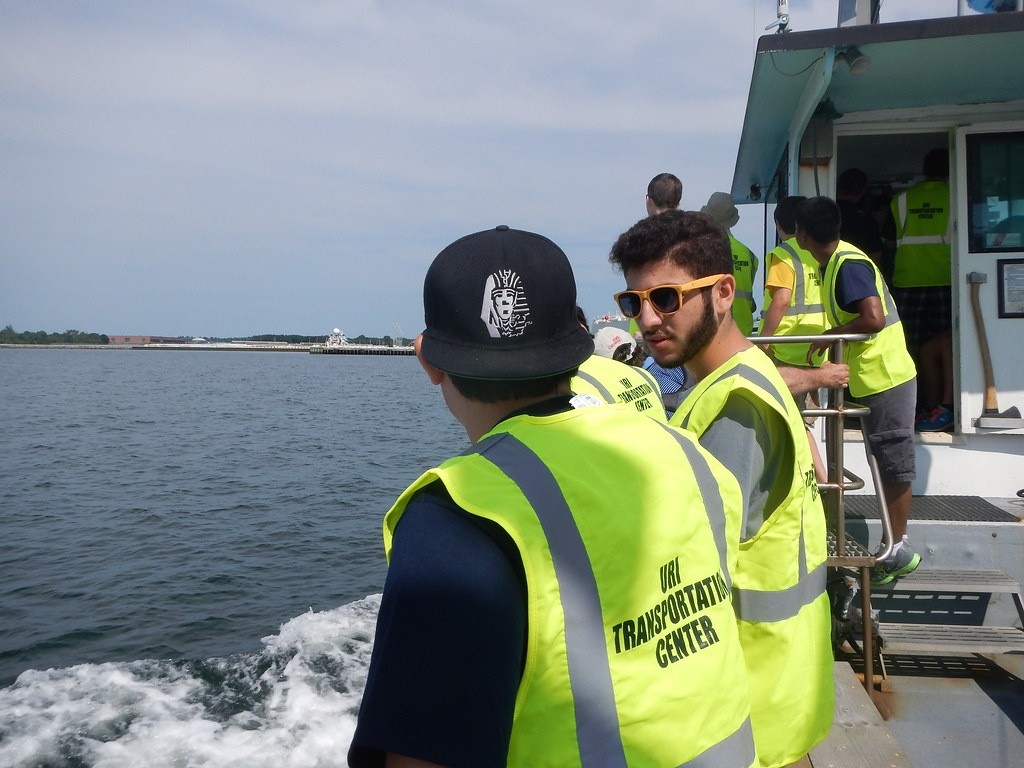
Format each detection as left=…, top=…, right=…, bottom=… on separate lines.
left=871, top=545, right=922, bottom=586
left=914, top=405, right=953, bottom=432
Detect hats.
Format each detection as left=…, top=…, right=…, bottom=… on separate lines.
left=590, top=327, right=636, bottom=364
left=419, top=224, right=595, bottom=381
left=699, top=192, right=743, bottom=229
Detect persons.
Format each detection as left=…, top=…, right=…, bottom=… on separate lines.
left=349, top=225, right=759, bottom=768
left=753, top=195, right=832, bottom=497
left=607, top=210, right=834, bottom=767
left=915, top=329, right=953, bottom=432
left=570, top=308, right=669, bottom=425
left=701, top=191, right=759, bottom=337
left=590, top=326, right=849, bottom=425
left=889, top=149, right=950, bottom=354
left=793, top=197, right=923, bottom=585
left=646, top=172, right=683, bottom=218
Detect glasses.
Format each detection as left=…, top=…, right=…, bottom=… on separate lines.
left=615, top=274, right=722, bottom=318
